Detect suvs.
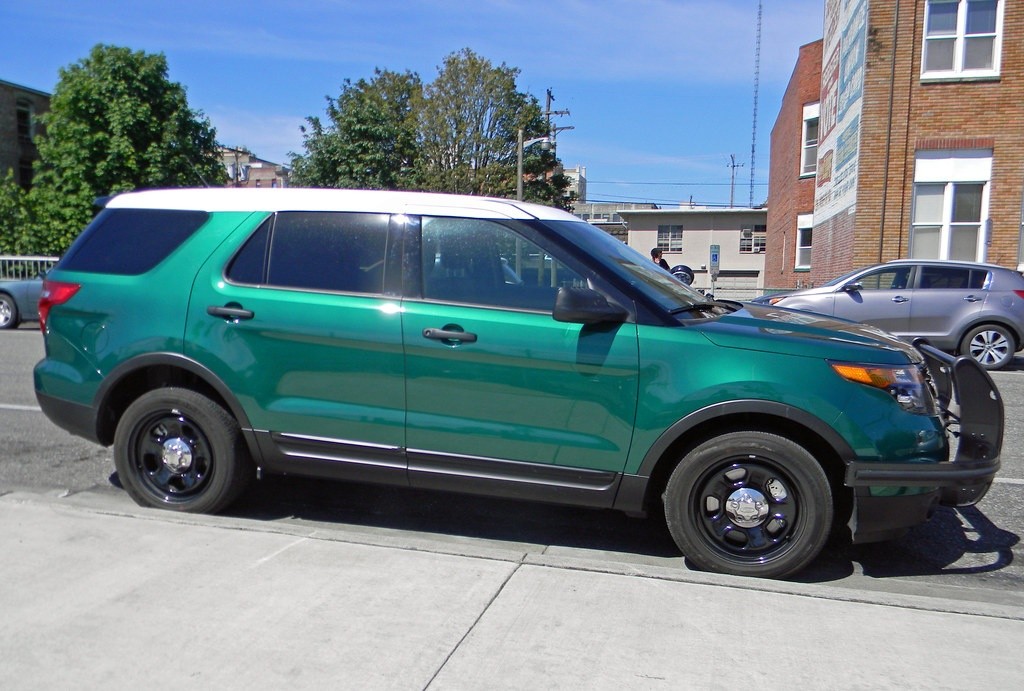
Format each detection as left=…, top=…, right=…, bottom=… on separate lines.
left=748, top=258, right=1023, bottom=372
left=33, top=187, right=1005, bottom=579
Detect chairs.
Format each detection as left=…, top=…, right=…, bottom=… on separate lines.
left=434, top=234, right=477, bottom=302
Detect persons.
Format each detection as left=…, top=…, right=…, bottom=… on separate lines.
left=649, top=248, right=670, bottom=273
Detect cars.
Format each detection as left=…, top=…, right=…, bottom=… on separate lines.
left=363, top=247, right=523, bottom=285
left=0, top=269, right=52, bottom=329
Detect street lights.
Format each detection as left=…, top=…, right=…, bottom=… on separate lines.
left=510, top=127, right=555, bottom=282
